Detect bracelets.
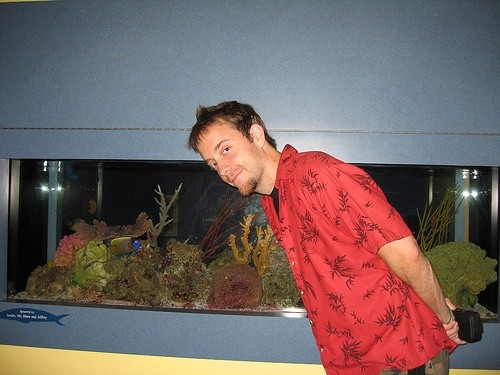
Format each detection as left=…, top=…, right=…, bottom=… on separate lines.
left=439, top=300, right=453, bottom=326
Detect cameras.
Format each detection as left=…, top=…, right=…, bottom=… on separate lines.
left=453, top=308, right=484, bottom=344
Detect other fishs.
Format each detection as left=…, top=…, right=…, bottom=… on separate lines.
left=105, top=237, right=144, bottom=259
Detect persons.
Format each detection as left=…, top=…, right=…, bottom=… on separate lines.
left=186, top=98, right=466, bottom=375
